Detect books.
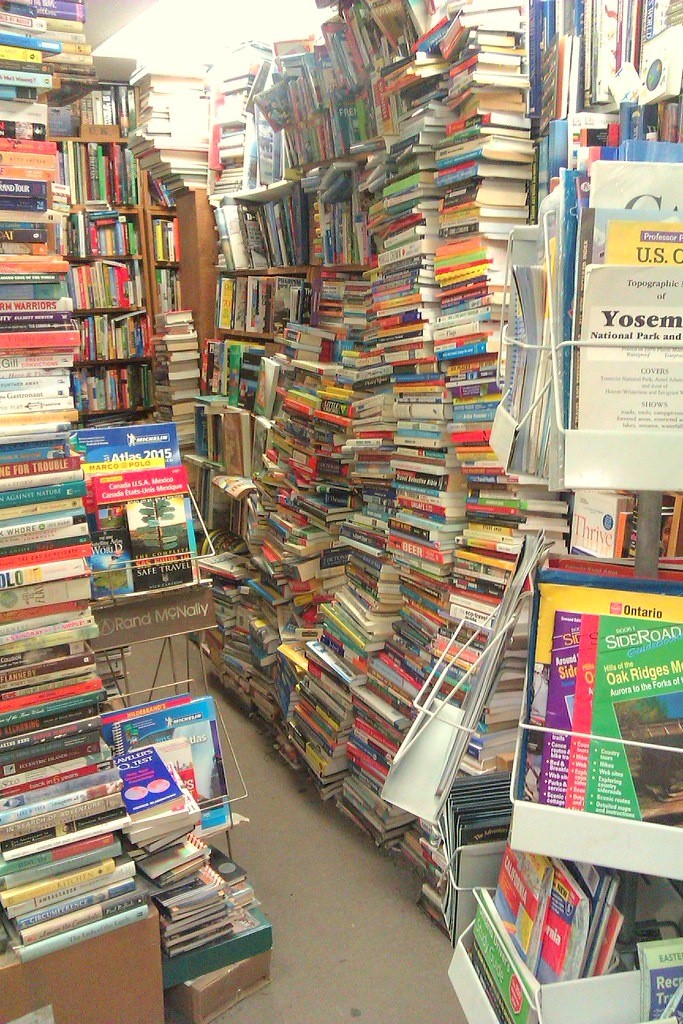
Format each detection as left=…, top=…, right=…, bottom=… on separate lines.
left=0, top=0, right=683, bottom=1024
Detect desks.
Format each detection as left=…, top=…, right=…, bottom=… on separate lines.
left=0, top=902, right=166, bottom=1024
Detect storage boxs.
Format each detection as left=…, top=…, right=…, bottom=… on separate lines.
left=168, top=946, right=271, bottom=1024
left=160, top=906, right=272, bottom=990
left=495, top=752, right=514, bottom=773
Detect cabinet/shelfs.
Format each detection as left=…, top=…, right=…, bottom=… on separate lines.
left=140, top=168, right=183, bottom=316
left=48, top=134, right=154, bottom=428
left=175, top=188, right=215, bottom=354
left=212, top=263, right=310, bottom=353
